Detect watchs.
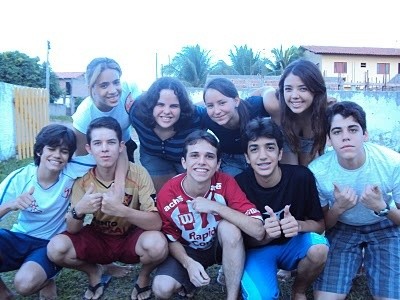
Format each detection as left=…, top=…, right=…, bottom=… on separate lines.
left=374, top=203, right=391, bottom=218
left=72, top=207, right=86, bottom=221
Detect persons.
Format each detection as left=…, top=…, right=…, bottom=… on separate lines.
left=233, top=117, right=330, bottom=300
left=0, top=124, right=130, bottom=300
left=308, top=101, right=400, bottom=300
left=151, top=131, right=265, bottom=300
left=73, top=57, right=337, bottom=298
left=47, top=116, right=170, bottom=300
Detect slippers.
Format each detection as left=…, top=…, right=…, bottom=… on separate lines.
left=130, top=274, right=152, bottom=300
left=177, top=284, right=196, bottom=298
left=83, top=274, right=112, bottom=300
